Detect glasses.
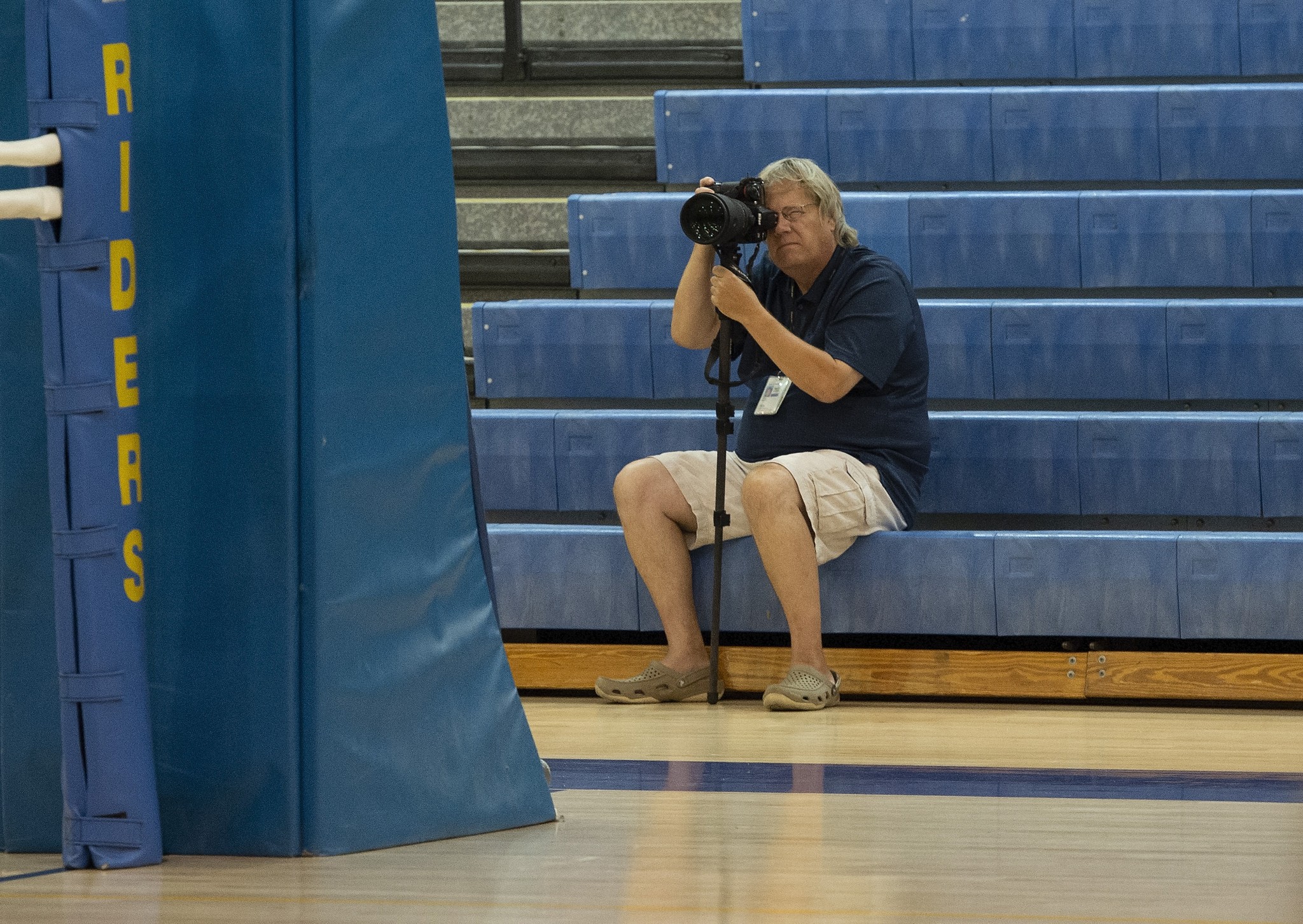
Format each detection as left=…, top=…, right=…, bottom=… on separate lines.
left=781, top=204, right=817, bottom=222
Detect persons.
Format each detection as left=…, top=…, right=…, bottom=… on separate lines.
left=587, top=158, right=931, bottom=712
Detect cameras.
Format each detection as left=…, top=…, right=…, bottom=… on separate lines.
left=679, top=174, right=778, bottom=248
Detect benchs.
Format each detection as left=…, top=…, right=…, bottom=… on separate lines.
left=401, top=1, right=1303, bottom=717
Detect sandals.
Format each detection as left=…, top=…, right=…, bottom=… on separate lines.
left=593, top=661, right=723, bottom=703
left=762, top=664, right=842, bottom=711
